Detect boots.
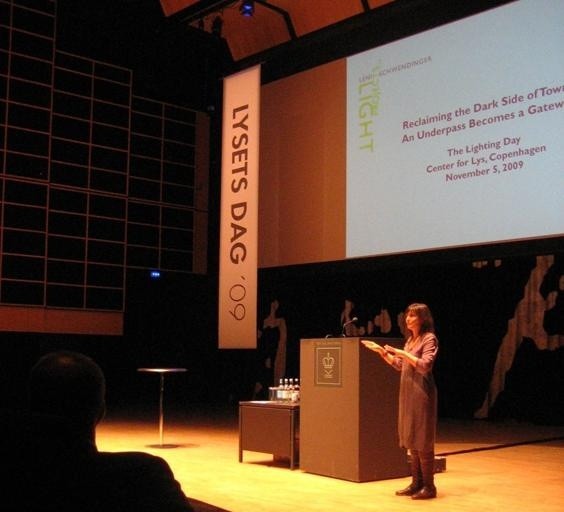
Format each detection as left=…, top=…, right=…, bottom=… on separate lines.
left=396, top=454, right=422, bottom=497
left=412, top=452, right=436, bottom=499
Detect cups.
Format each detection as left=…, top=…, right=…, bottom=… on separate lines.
left=268, top=386, right=277, bottom=401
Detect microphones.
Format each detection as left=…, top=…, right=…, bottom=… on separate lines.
left=343, top=317, right=358, bottom=327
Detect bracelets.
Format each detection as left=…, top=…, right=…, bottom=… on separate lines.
left=380, top=352, right=387, bottom=358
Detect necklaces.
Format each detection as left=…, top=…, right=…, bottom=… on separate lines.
left=409, top=334, right=420, bottom=351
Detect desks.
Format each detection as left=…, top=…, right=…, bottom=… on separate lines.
left=240, top=399, right=301, bottom=470
left=140, top=365, right=189, bottom=447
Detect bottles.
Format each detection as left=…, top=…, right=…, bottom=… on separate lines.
left=277, top=378, right=299, bottom=404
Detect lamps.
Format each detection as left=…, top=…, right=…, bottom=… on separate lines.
left=238, top=1, right=256, bottom=18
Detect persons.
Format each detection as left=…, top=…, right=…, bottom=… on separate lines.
left=0, top=349, right=193, bottom=507
left=360, top=300, right=441, bottom=500
